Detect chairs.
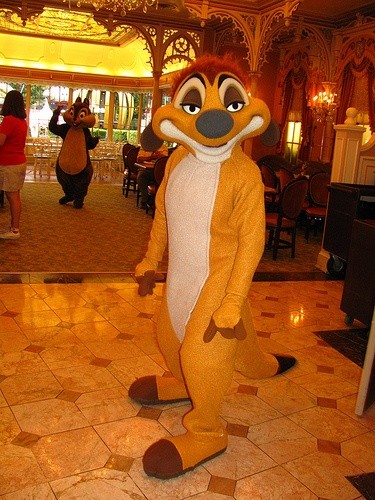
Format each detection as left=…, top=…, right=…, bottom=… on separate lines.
left=306, top=171, right=330, bottom=241
left=279, top=166, right=310, bottom=234
left=120, top=142, right=138, bottom=199
left=260, top=162, right=280, bottom=209
left=146, top=154, right=172, bottom=216
left=32, top=137, right=127, bottom=183
left=265, top=176, right=311, bottom=261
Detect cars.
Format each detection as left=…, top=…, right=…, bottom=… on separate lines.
left=51, top=100, right=68, bottom=111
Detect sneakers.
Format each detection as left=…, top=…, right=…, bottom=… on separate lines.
left=0, top=227, right=11, bottom=234
left=0, top=231, right=20, bottom=239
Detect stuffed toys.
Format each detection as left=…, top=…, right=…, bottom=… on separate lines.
left=127, top=51, right=298, bottom=479
left=49, top=96, right=99, bottom=209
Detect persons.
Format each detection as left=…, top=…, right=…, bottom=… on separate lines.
left=0, top=90, right=27, bottom=240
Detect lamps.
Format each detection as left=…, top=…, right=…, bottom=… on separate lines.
left=309, top=88, right=338, bottom=124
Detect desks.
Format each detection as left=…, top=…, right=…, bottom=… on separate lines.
left=264, top=183, right=278, bottom=208
left=134, top=162, right=155, bottom=206
